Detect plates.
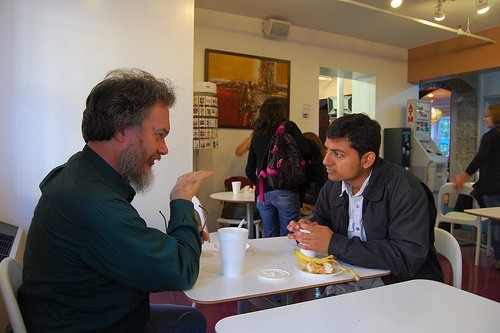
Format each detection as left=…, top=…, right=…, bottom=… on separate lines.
left=214, top=241, right=250, bottom=252
left=292, top=262, right=343, bottom=278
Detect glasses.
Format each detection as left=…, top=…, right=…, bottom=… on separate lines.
left=160, top=205, right=208, bottom=236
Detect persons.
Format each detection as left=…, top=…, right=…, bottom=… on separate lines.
left=289, top=114, right=445, bottom=298
left=235, top=98, right=313, bottom=304
left=17, top=68, right=214, bottom=333
left=455, top=104, right=500, bottom=269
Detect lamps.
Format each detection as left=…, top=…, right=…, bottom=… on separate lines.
left=476, top=0, right=490, bottom=14
left=433, top=0, right=445, bottom=21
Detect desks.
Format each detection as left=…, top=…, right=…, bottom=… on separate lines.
left=214, top=279, right=500, bottom=333
left=464, top=206, right=500, bottom=221
left=210, top=191, right=257, bottom=239
left=183, top=236, right=391, bottom=308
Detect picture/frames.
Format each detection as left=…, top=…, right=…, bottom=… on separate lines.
left=205, top=49, right=292, bottom=129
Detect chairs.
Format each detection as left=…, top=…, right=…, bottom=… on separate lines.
left=434, top=227, right=462, bottom=290
left=435, top=182, right=491, bottom=266
left=217, top=176, right=262, bottom=239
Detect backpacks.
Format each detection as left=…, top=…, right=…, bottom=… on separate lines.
left=258, top=120, right=307, bottom=202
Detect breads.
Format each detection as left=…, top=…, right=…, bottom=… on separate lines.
left=307, top=262, right=335, bottom=274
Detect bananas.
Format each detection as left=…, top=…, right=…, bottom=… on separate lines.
left=293, top=249, right=334, bottom=267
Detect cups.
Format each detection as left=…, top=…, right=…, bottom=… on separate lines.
left=217, top=227, right=248, bottom=278
left=299, top=228, right=317, bottom=258
left=232, top=181, right=241, bottom=193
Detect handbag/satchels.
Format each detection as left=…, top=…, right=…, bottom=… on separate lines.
left=221, top=180, right=261, bottom=234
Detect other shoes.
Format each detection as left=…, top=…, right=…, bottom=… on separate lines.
left=493, top=262, right=500, bottom=272
